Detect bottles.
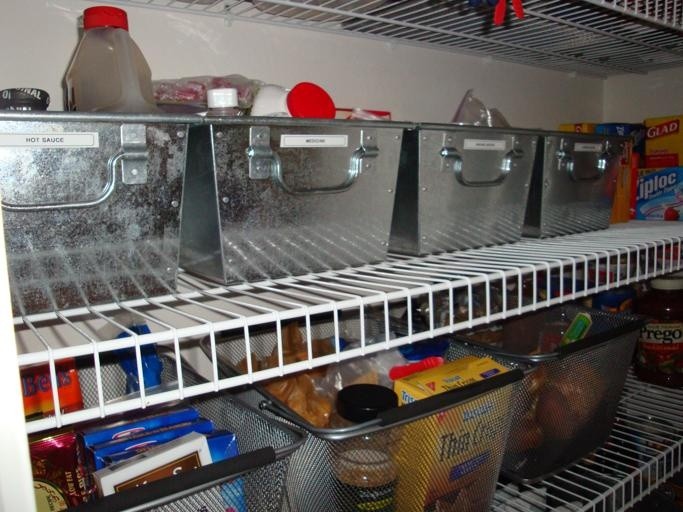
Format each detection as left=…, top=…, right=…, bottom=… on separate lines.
left=250, top=83, right=337, bottom=120
left=207, top=86, right=242, bottom=117
left=635, top=277, right=682, bottom=386
left=331, top=384, right=400, bottom=512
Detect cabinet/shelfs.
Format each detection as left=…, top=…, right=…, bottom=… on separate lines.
left=0, top=0, right=683, bottom=512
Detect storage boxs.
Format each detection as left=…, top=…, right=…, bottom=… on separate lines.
left=388, top=123, right=549, bottom=257
left=522, top=131, right=631, bottom=238
left=179, top=115, right=417, bottom=286
left=55, top=345, right=308, bottom=512
left=199, top=307, right=524, bottom=512
left=396, top=294, right=651, bottom=484
left=0, top=110, right=203, bottom=319
